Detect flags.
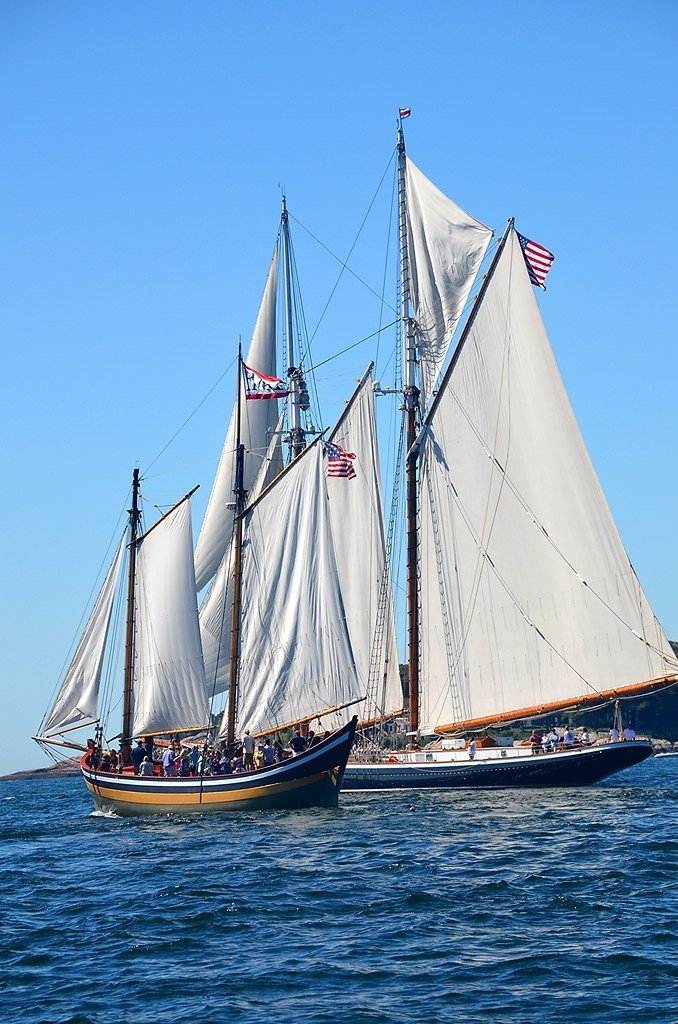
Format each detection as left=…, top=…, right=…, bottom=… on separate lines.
left=325, top=440, right=357, bottom=481
left=241, top=361, right=289, bottom=400
left=515, top=230, right=555, bottom=290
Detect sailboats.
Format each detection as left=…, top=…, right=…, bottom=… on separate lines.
left=30, top=194, right=388, bottom=819
left=338, top=105, right=676, bottom=792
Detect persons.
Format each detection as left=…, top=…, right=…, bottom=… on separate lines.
left=579, top=728, right=589, bottom=747
left=608, top=725, right=619, bottom=743
left=262, top=739, right=276, bottom=767
left=529, top=730, right=542, bottom=754
left=563, top=725, right=574, bottom=749
left=288, top=730, right=308, bottom=757
left=352, top=739, right=383, bottom=762
left=138, top=756, right=156, bottom=776
left=172, top=748, right=192, bottom=776
left=541, top=732, right=550, bottom=753
left=622, top=723, right=636, bottom=741
left=271, top=740, right=284, bottom=764
left=206, top=730, right=214, bottom=748
left=90, top=743, right=120, bottom=773
left=130, top=740, right=149, bottom=775
left=237, top=730, right=255, bottom=772
left=161, top=745, right=176, bottom=777
left=305, top=731, right=320, bottom=748
left=550, top=729, right=559, bottom=752
left=188, top=745, right=242, bottom=776
left=467, top=737, right=477, bottom=760
left=323, top=731, right=331, bottom=741
